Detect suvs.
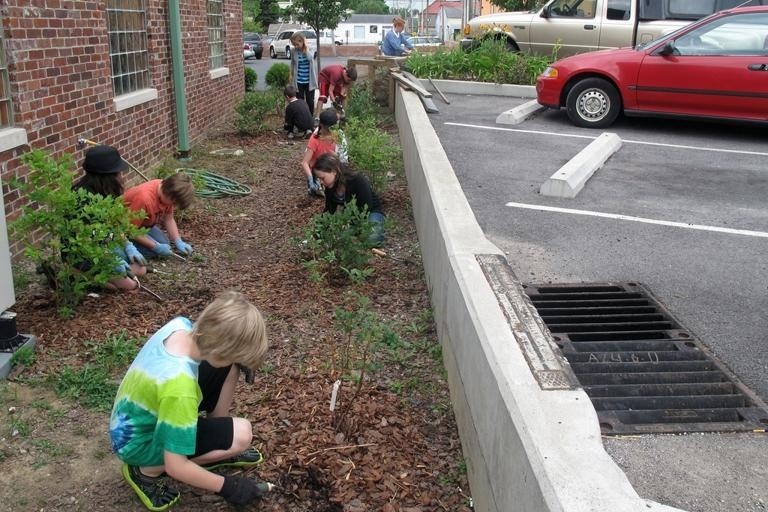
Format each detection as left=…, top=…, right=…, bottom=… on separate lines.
left=268, top=29, right=318, bottom=59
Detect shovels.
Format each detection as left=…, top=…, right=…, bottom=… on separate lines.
left=256, top=470, right=309, bottom=495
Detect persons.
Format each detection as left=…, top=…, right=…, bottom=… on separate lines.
left=108, top=289, right=269, bottom=512
left=313, top=63, right=357, bottom=119
left=41, top=144, right=148, bottom=293
left=305, top=107, right=349, bottom=164
left=380, top=16, right=417, bottom=56
left=287, top=30, right=320, bottom=117
left=299, top=109, right=337, bottom=197
left=310, top=151, right=386, bottom=250
left=282, top=86, right=314, bottom=139
left=118, top=172, right=195, bottom=259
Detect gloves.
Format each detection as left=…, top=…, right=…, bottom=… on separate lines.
left=308, top=176, right=321, bottom=194
left=215, top=475, right=263, bottom=508
left=124, top=241, right=148, bottom=266
left=107, top=252, right=131, bottom=278
left=174, top=239, right=194, bottom=256
left=235, top=362, right=255, bottom=385
left=151, top=242, right=172, bottom=257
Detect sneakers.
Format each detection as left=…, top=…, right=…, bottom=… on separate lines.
left=122, top=463, right=181, bottom=512
left=313, top=118, right=320, bottom=127
left=301, top=129, right=312, bottom=139
left=201, top=445, right=264, bottom=471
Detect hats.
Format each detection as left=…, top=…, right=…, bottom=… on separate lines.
left=81, top=145, right=128, bottom=174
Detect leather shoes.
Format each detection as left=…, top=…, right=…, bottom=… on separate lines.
left=36, top=260, right=62, bottom=290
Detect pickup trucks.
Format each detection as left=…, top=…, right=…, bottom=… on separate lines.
left=310, top=30, right=344, bottom=46
left=459, top=0, right=768, bottom=55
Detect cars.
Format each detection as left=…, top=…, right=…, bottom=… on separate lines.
left=243, top=32, right=264, bottom=60
left=528, top=3, right=768, bottom=136
left=243, top=42, right=255, bottom=61
left=405, top=37, right=444, bottom=47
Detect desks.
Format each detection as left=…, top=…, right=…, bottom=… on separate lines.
left=341, top=54, right=426, bottom=112
